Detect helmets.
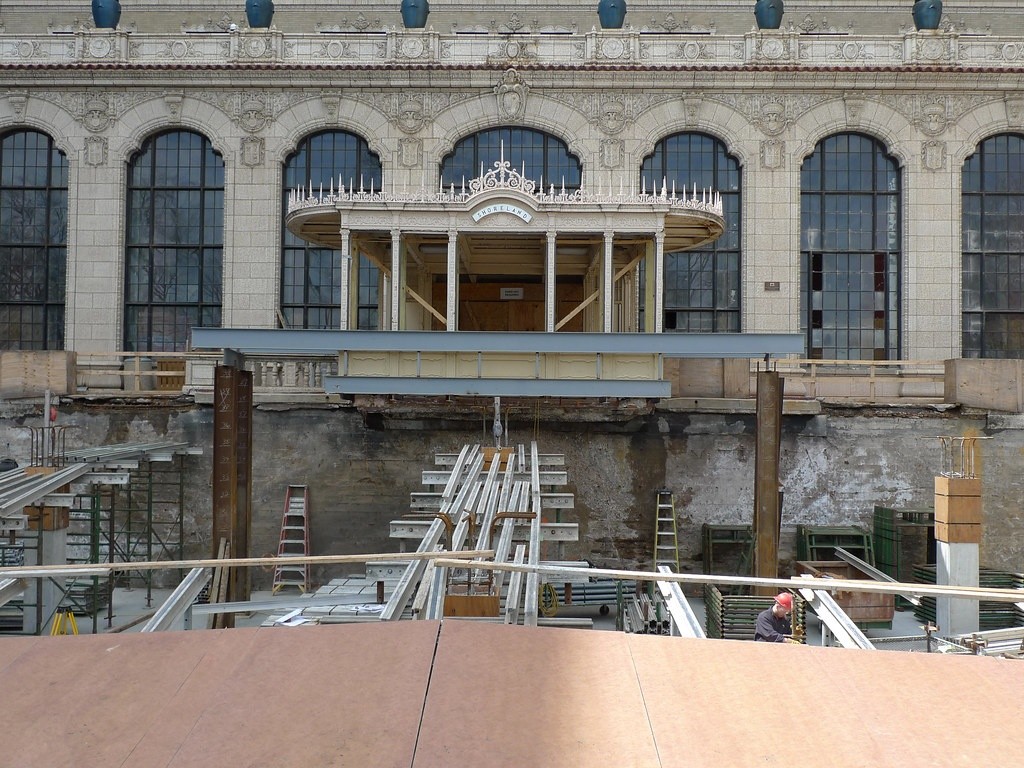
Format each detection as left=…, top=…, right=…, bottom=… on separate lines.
left=773, top=593, right=792, bottom=612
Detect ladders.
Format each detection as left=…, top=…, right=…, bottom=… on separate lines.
left=650, top=488, right=683, bottom=598
left=271, top=483, right=312, bottom=596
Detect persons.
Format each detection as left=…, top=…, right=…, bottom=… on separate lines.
left=755, top=592, right=805, bottom=645
